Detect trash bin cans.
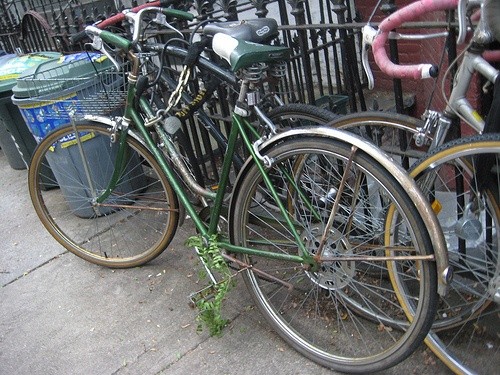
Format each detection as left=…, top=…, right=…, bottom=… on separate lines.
left=1, top=51, right=65, bottom=192
left=0, top=54, right=27, bottom=171
left=11, top=50, right=148, bottom=220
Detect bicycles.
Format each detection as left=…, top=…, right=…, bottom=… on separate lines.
left=19, top=0, right=500, bottom=375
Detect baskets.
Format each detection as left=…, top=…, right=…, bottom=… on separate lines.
left=21, top=53, right=157, bottom=120
